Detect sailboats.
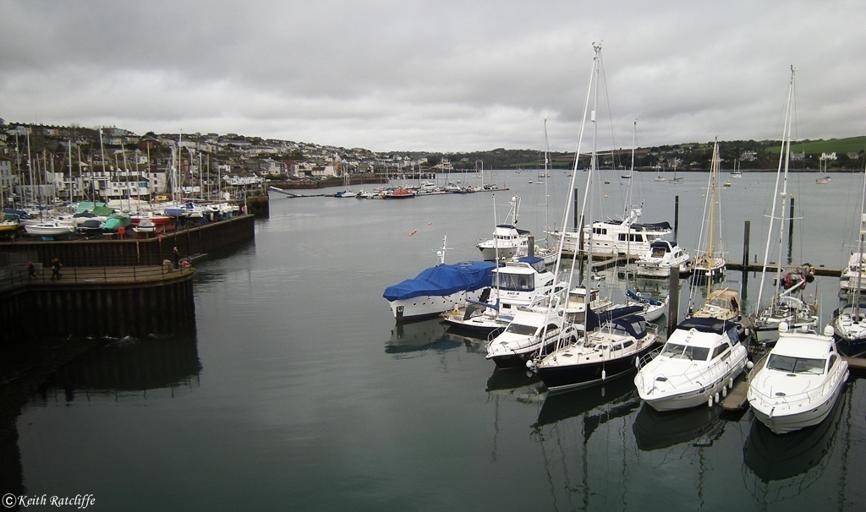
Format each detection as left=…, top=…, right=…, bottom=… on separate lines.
left=542, top=119, right=677, bottom=261
left=0, top=115, right=267, bottom=239
left=329, top=153, right=510, bottom=201
left=527, top=38, right=671, bottom=397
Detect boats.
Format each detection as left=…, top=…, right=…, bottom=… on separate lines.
left=380, top=234, right=547, bottom=325
left=473, top=189, right=540, bottom=262
left=481, top=283, right=580, bottom=370
left=744, top=319, right=852, bottom=437
left=440, top=190, right=572, bottom=349
left=632, top=314, right=757, bottom=415
left=824, top=165, right=866, bottom=364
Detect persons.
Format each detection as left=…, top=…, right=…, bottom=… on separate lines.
left=26, top=261, right=40, bottom=281
left=171, top=247, right=180, bottom=269
left=50, top=258, right=61, bottom=281
